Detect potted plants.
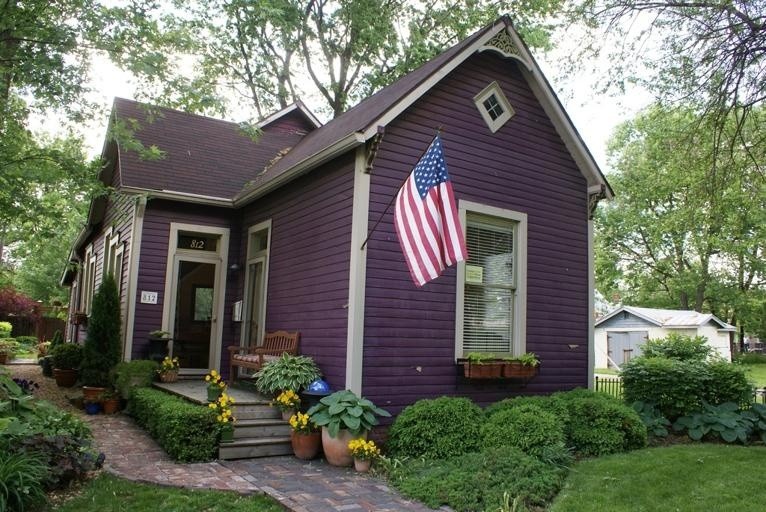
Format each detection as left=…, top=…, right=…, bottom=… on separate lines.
left=99, top=391, right=119, bottom=414
left=84, top=398, right=98, bottom=416
left=505, top=352, right=542, bottom=378
left=146, top=331, right=173, bottom=361
left=464, top=351, right=505, bottom=378
left=52, top=343, right=83, bottom=387
left=310, top=390, right=391, bottom=467
left=110, top=360, right=159, bottom=400
left=81, top=273, right=122, bottom=404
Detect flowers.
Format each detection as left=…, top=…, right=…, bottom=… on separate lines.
left=270, top=390, right=299, bottom=409
left=205, top=370, right=225, bottom=388
left=210, top=392, right=236, bottom=425
left=289, top=411, right=317, bottom=433
left=161, top=356, right=174, bottom=370
left=348, top=437, right=380, bottom=458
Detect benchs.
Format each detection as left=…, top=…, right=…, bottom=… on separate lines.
left=228, top=331, right=300, bottom=386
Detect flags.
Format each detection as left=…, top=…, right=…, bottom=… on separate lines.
left=394, top=133, right=469, bottom=288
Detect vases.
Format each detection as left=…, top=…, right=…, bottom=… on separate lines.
left=354, top=458, right=370, bottom=472
left=161, top=371, right=173, bottom=380
left=221, top=428, right=235, bottom=442
left=280, top=409, right=293, bottom=419
left=208, top=388, right=221, bottom=401
left=292, top=435, right=318, bottom=460
